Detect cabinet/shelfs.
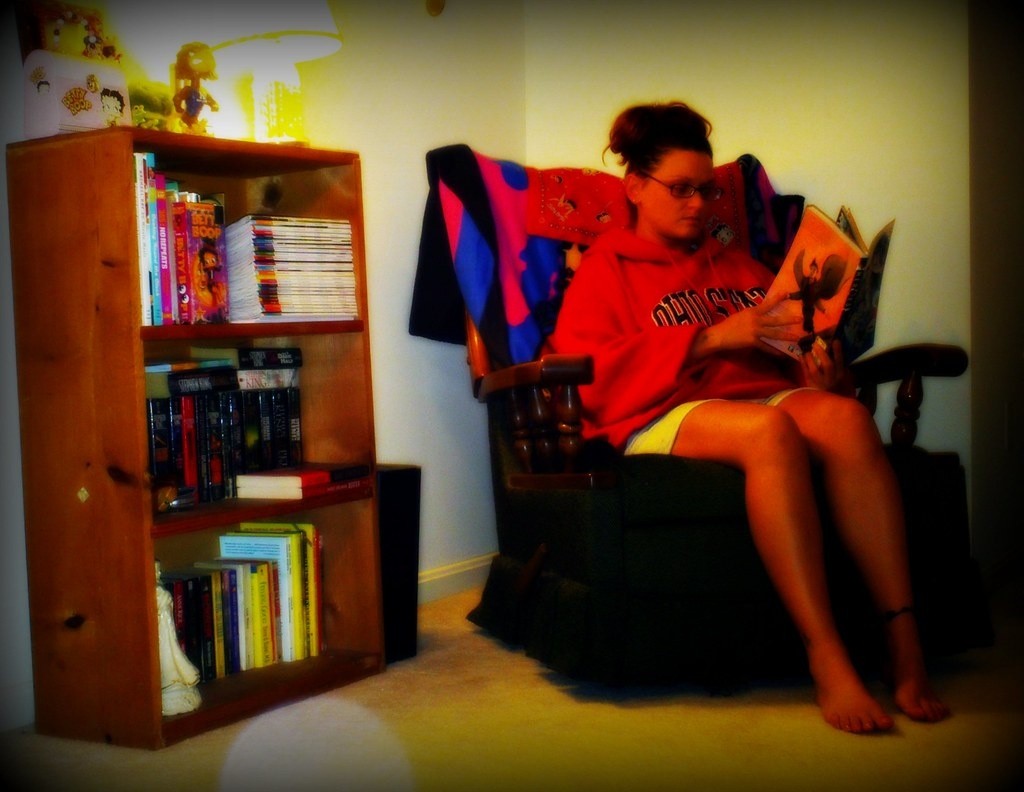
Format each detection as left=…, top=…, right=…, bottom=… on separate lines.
left=4, top=126, right=390, bottom=749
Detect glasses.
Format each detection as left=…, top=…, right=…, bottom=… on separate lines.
left=635, top=169, right=723, bottom=202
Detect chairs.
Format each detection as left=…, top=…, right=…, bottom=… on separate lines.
left=424, top=145, right=974, bottom=699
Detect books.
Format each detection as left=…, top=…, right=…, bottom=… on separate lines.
left=225, top=215, right=357, bottom=322
left=145, top=347, right=374, bottom=513
left=134, top=149, right=231, bottom=328
left=162, top=519, right=328, bottom=681
left=757, top=205, right=894, bottom=368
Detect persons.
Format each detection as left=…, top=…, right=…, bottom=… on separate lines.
left=153, top=559, right=202, bottom=717
left=540, top=103, right=950, bottom=734
